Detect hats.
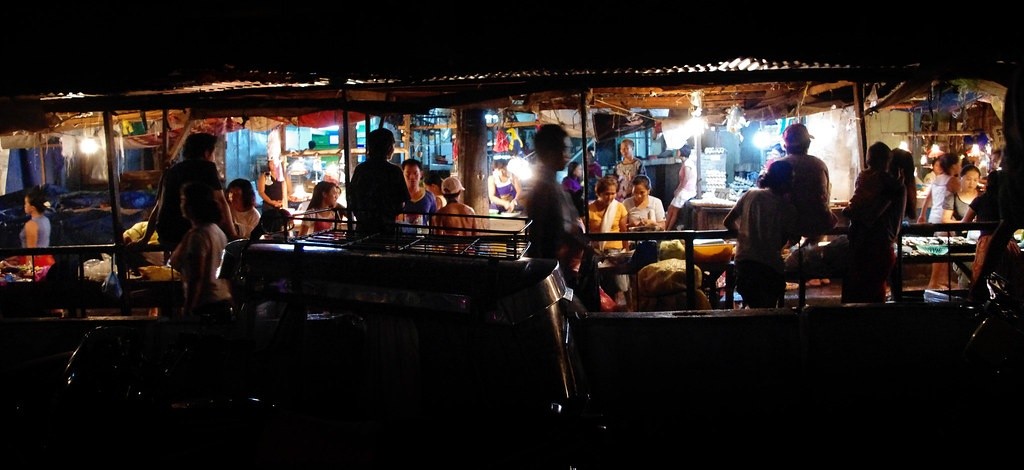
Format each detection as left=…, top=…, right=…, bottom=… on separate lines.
left=782, top=124, right=815, bottom=146
left=442, top=178, right=465, bottom=195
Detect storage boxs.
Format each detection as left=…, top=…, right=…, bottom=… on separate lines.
left=681, top=243, right=735, bottom=262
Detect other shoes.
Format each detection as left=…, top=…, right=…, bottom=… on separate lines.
left=805, top=279, right=830, bottom=287
left=926, top=285, right=948, bottom=291
left=785, top=281, right=799, bottom=290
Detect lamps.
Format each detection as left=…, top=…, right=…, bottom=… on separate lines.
left=899, top=133, right=984, bottom=159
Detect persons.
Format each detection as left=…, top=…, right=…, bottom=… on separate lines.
left=0, top=193, right=56, bottom=282
left=123, top=127, right=479, bottom=325
left=486, top=122, right=1020, bottom=312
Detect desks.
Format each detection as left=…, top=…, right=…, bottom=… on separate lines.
left=0, top=278, right=180, bottom=320
left=703, top=251, right=976, bottom=311
